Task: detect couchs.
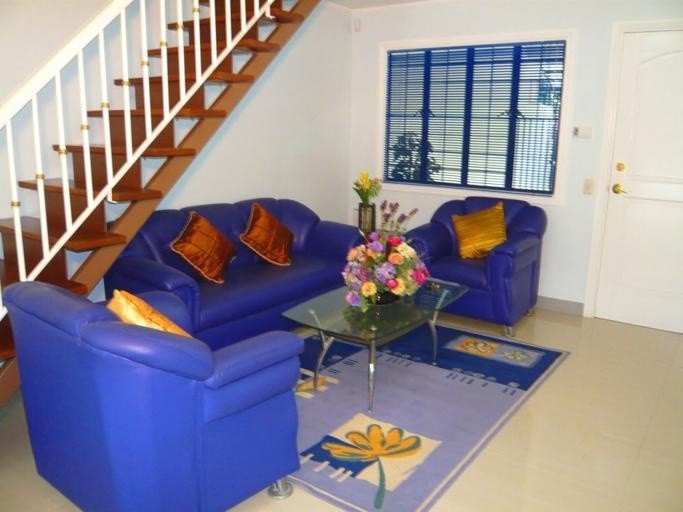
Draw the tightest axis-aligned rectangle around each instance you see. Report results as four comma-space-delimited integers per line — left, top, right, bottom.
102, 198, 364, 351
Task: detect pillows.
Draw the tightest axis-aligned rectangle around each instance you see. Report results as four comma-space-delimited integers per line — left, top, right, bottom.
451, 201, 507, 259
170, 211, 239, 284
238, 202, 295, 266
106, 288, 194, 338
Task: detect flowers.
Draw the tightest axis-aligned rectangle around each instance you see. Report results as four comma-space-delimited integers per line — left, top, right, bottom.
353, 171, 383, 204
340, 200, 430, 312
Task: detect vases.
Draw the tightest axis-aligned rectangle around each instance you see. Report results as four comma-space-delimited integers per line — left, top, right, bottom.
358, 202, 376, 234
366, 291, 400, 304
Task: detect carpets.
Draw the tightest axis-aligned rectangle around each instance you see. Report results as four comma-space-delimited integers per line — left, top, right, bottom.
287, 317, 571, 512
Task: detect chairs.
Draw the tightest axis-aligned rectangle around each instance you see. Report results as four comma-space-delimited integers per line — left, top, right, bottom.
402, 196, 546, 337
2, 281, 305, 512
